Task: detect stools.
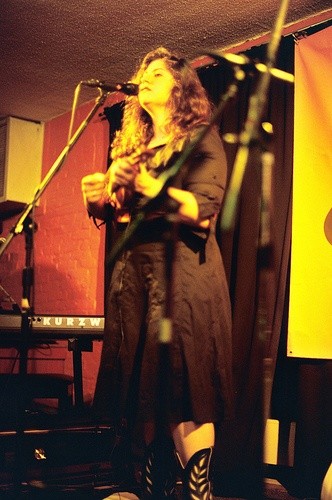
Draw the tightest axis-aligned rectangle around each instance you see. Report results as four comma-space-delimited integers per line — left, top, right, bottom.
5, 376, 73, 499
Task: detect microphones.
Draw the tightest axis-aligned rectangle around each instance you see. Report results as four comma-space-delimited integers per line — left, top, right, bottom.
80, 79, 139, 96
199, 50, 294, 83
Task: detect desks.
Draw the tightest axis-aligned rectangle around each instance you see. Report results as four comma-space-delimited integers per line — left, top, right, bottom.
0, 314, 109, 424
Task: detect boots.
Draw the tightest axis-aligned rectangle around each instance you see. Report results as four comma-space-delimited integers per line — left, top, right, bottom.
173, 448, 212, 500
133, 442, 167, 500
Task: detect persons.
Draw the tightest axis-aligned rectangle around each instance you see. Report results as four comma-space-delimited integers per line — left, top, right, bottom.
82, 48, 228, 500
